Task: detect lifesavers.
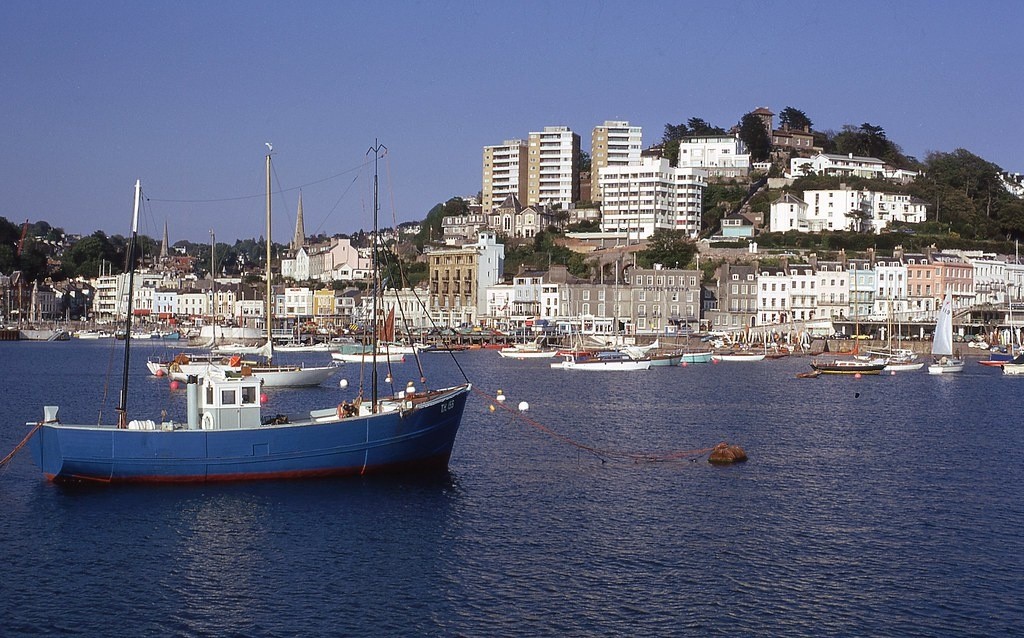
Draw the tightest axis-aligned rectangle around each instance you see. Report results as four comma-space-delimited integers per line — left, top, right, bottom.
201, 412, 213, 430
1001, 365, 1004, 370
170, 362, 179, 372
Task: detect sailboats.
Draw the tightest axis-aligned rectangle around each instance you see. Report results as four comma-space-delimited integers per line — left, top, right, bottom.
53, 143, 471, 389
22, 138, 475, 486
496, 264, 1024, 376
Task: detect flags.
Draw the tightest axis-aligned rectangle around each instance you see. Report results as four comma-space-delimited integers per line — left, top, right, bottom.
379, 305, 395, 343
